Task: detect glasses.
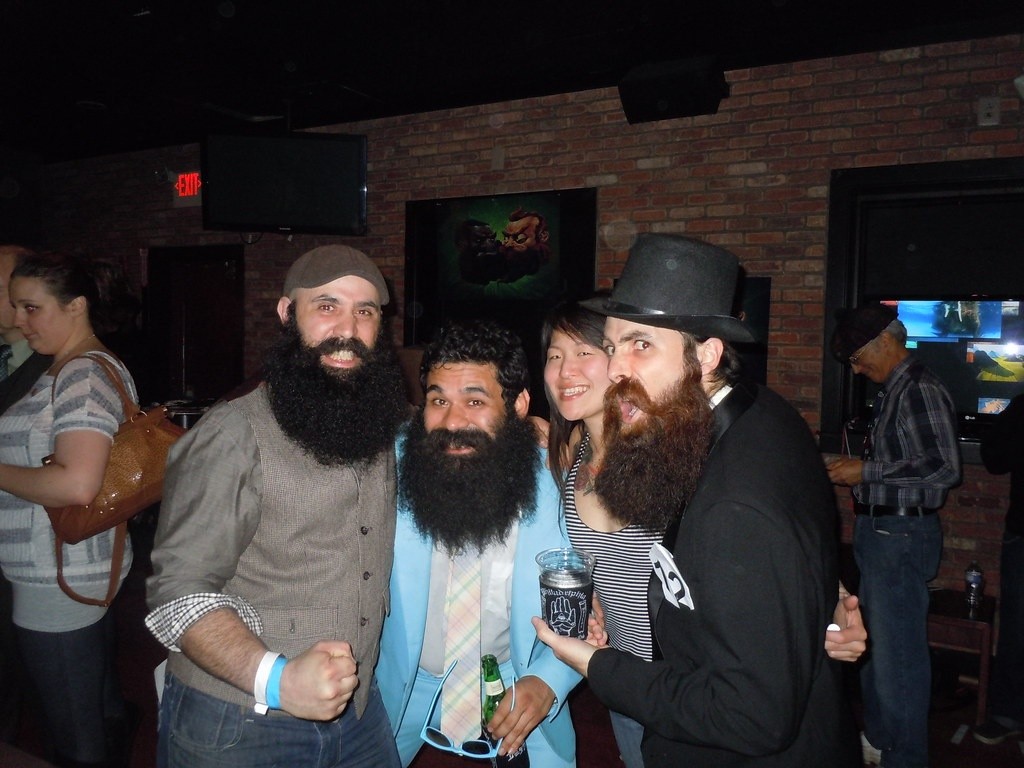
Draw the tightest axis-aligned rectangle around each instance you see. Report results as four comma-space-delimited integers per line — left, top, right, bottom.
419, 660, 516, 760
849, 340, 872, 365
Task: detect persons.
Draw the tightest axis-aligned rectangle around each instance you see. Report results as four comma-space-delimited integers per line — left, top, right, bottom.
0, 242, 132, 768
374, 234, 1024, 768
144, 243, 550, 768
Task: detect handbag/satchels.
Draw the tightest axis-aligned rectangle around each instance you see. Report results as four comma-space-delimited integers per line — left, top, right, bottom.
41, 353, 193, 608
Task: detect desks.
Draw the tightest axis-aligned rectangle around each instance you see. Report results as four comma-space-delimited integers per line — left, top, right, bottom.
928, 589, 997, 727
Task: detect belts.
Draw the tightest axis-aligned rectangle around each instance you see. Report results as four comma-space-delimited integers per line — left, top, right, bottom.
852, 499, 939, 519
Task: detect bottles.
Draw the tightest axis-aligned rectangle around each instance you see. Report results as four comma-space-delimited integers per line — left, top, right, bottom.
963, 559, 985, 606
481, 654, 530, 768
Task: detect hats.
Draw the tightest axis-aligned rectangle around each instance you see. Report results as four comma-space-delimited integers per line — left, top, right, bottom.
576, 231, 757, 345
830, 302, 898, 365
281, 244, 390, 307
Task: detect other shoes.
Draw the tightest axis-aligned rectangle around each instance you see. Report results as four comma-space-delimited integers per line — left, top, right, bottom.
974, 718, 1024, 751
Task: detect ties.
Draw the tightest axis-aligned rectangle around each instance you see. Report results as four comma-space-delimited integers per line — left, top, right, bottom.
0, 345, 13, 384
441, 531, 482, 757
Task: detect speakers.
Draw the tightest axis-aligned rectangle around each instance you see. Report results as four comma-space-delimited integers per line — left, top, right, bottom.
618, 54, 726, 125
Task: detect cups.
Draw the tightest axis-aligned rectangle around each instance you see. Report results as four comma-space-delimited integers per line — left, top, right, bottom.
536, 547, 595, 641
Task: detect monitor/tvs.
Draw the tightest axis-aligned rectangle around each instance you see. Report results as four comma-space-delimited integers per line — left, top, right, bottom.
200, 130, 368, 238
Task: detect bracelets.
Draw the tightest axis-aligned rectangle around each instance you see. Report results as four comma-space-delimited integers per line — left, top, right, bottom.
254, 649, 289, 716
